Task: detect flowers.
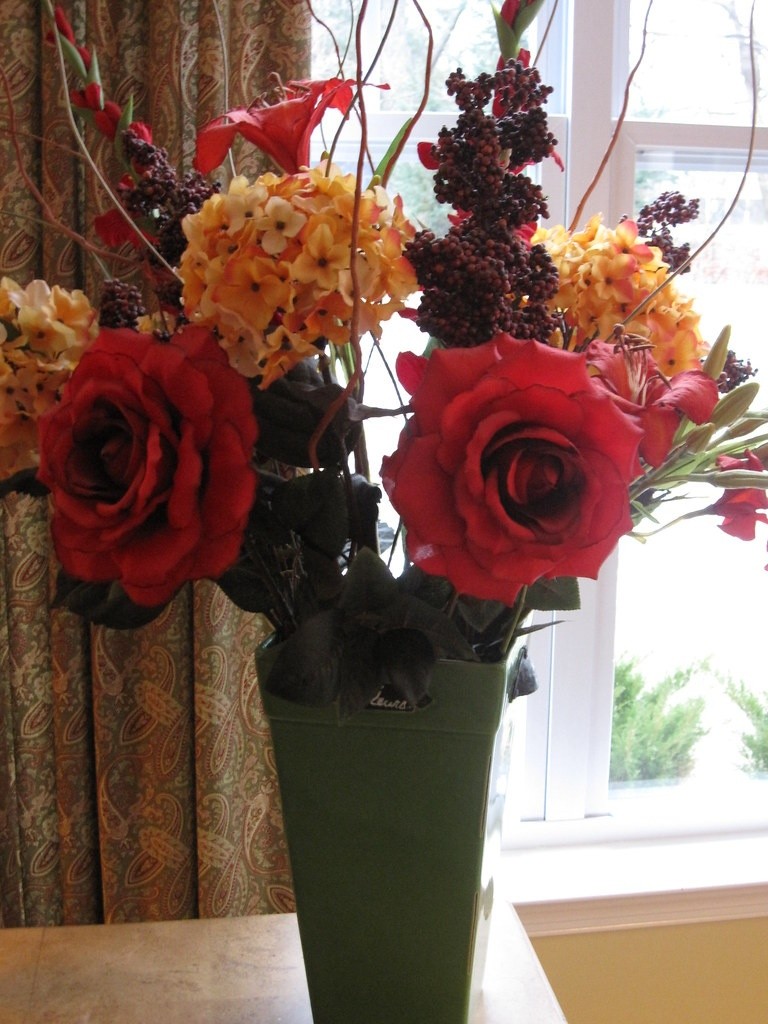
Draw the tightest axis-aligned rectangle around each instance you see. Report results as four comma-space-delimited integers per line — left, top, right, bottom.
0, 0, 768, 711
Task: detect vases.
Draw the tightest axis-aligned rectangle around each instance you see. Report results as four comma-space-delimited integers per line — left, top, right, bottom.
254, 631, 516, 1024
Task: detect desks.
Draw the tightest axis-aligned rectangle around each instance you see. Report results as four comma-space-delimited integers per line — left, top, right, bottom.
0, 899, 572, 1024
